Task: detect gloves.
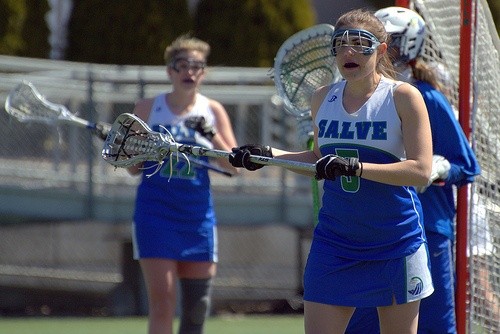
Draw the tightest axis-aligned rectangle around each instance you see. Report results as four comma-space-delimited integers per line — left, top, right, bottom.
184, 115, 217, 138
413, 155, 449, 192
229, 143, 273, 170
314, 153, 359, 180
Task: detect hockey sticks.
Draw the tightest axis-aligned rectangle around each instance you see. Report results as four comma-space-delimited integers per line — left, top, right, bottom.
274, 24, 344, 228
100, 112, 340, 176
4, 81, 233, 176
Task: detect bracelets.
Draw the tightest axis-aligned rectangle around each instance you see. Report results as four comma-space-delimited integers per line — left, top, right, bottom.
358, 163, 363, 177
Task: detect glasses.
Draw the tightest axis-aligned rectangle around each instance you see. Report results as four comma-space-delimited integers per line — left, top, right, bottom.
330, 28, 380, 55
169, 58, 205, 76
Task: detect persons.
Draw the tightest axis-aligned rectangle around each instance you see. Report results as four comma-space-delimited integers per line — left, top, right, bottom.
229, 8, 435, 334
125, 34, 241, 334
343, 7, 481, 334
0, 0, 324, 181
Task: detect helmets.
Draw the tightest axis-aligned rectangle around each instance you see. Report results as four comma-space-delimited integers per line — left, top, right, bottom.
374, 6, 425, 62
